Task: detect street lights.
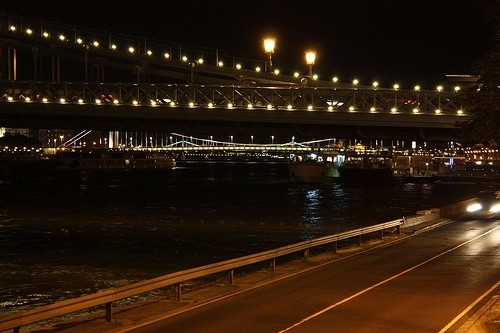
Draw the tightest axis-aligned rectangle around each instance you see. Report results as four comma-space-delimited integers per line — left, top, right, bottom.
306, 51, 315, 80
263, 39, 275, 74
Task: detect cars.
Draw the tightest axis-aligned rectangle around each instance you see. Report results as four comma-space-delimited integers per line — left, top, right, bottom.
468, 194, 500, 219
